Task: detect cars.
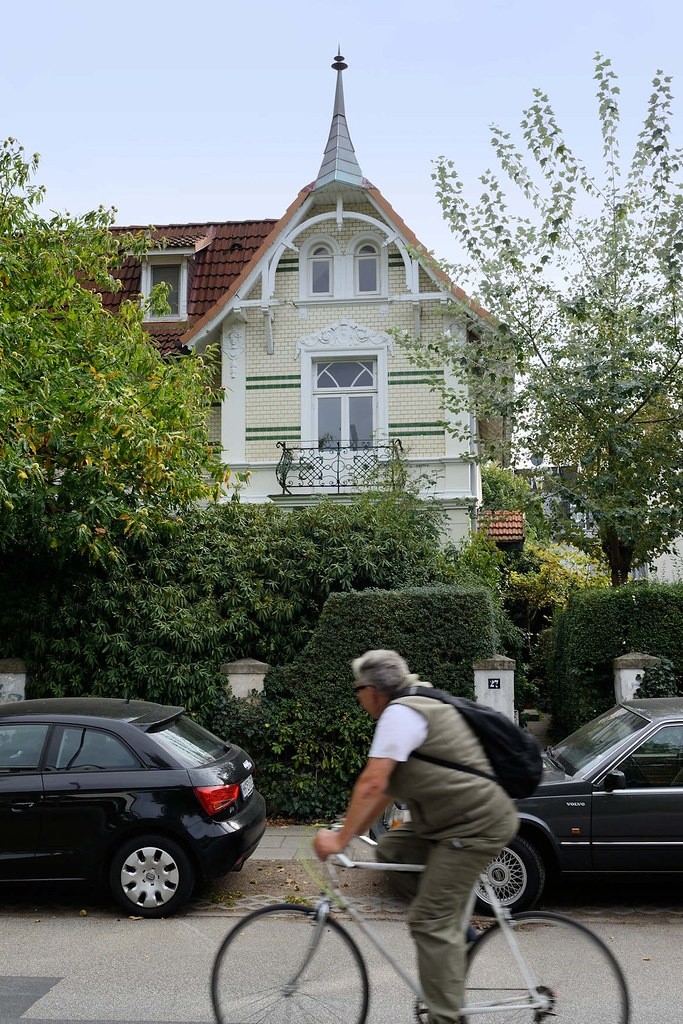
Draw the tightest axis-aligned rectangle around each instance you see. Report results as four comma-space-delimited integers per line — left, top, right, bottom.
369, 694, 683, 918
0, 697, 269, 917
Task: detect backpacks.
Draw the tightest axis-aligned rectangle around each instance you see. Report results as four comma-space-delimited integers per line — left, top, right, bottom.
388, 686, 543, 799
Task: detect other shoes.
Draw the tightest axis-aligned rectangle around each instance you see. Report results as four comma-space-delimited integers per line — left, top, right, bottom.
464, 926, 475, 944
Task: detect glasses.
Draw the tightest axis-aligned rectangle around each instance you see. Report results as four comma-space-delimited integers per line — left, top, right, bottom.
352, 684, 378, 695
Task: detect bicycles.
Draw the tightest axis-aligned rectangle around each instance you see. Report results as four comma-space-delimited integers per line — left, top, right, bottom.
211, 824, 631, 1024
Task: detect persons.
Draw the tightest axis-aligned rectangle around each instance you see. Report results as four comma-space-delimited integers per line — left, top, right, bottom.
312, 651, 517, 1024
